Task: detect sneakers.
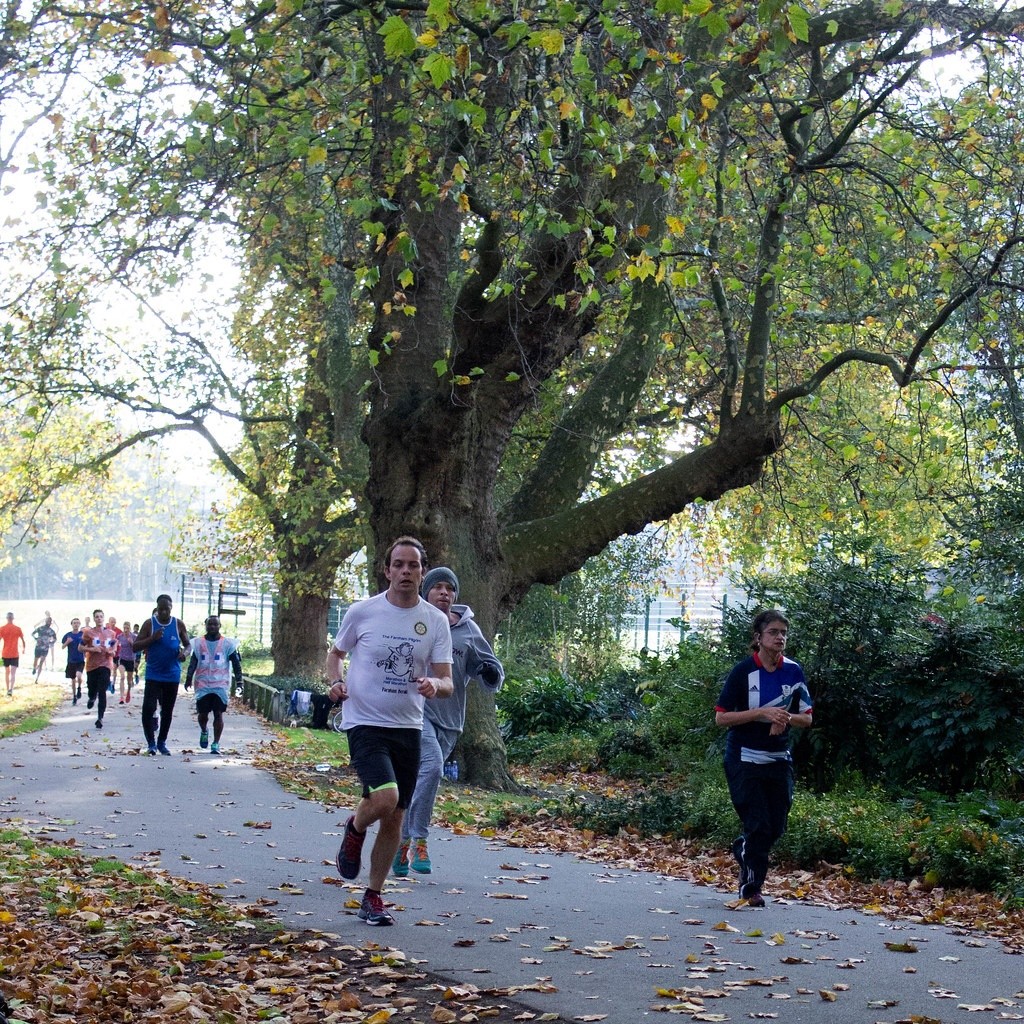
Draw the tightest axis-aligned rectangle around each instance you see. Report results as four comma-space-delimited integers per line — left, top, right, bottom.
748, 892, 765, 906
146, 745, 156, 753
199, 730, 208, 749
357, 892, 397, 926
392, 841, 409, 878
210, 742, 220, 753
409, 838, 431, 874
336, 814, 366, 881
157, 742, 170, 754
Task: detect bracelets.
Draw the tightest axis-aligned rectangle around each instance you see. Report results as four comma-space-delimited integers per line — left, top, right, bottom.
330, 679, 343, 685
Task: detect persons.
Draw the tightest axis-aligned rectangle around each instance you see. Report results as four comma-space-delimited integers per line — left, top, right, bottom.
184, 615, 244, 754
0, 612, 25, 698
32, 607, 160, 729
714, 610, 814, 905
326, 536, 454, 925
132, 595, 191, 755
391, 567, 505, 876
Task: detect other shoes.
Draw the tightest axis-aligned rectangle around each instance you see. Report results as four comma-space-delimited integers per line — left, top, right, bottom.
77, 688, 81, 698
108, 681, 111, 691
135, 675, 139, 684
111, 686, 114, 693
7, 690, 11, 695
72, 696, 77, 705
88, 700, 94, 708
126, 694, 130, 703
95, 720, 103, 728
119, 697, 124, 703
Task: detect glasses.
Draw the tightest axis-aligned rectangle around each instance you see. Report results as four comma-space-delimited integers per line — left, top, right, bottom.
760, 629, 787, 637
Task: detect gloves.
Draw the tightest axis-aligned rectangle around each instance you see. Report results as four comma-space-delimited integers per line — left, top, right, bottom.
479, 663, 502, 686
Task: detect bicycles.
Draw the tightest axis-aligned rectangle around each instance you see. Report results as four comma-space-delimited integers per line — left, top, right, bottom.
332, 710, 347, 734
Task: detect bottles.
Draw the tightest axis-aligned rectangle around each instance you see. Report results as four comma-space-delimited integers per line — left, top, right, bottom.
236, 687, 242, 698
445, 760, 458, 781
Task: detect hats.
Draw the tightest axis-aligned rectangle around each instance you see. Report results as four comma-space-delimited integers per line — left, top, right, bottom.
423, 567, 459, 602
